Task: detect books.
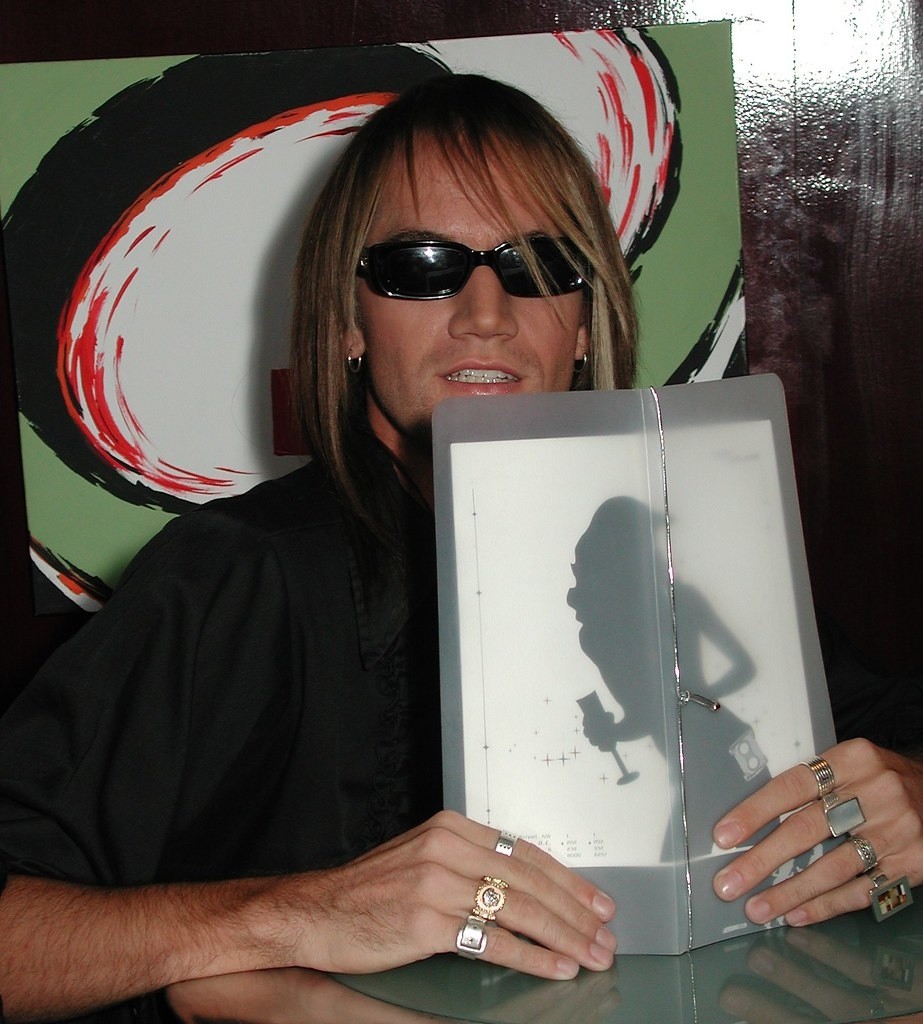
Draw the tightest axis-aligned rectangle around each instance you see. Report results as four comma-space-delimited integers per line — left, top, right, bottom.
433, 374, 849, 956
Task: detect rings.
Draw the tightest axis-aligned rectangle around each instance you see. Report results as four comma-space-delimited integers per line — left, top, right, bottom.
471, 876, 509, 921
872, 945, 914, 991
869, 997, 883, 1020
821, 793, 866, 838
848, 833, 878, 873
798, 756, 834, 797
456, 915, 486, 960
867, 867, 913, 922
495, 831, 519, 856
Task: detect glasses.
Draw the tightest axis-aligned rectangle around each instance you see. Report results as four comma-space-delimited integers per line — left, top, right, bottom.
352, 234, 592, 301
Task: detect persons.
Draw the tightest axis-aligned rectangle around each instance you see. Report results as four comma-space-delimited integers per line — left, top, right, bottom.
1, 76, 923, 1024
